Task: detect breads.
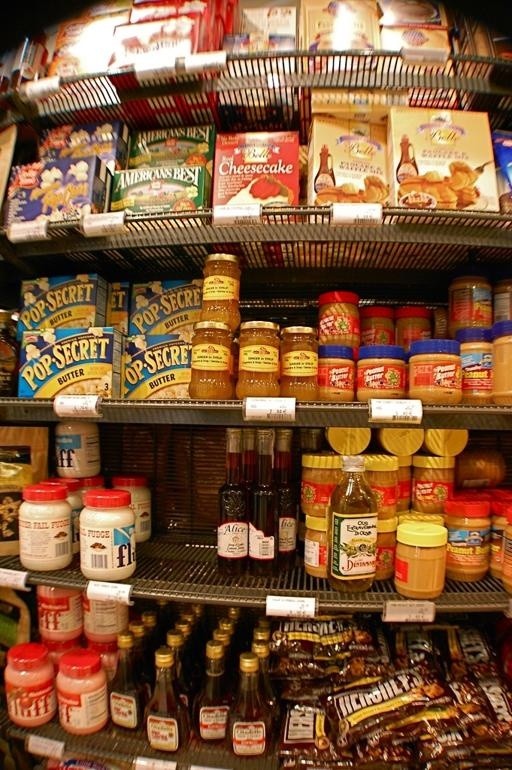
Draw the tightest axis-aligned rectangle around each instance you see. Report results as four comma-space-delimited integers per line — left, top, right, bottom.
398, 162, 477, 208
317, 177, 389, 205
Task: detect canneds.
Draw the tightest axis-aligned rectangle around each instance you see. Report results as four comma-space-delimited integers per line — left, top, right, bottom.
493, 279, 512, 323
201, 254, 242, 331
447, 276, 493, 340
189, 321, 234, 401
236, 320, 280, 401
280, 326, 319, 404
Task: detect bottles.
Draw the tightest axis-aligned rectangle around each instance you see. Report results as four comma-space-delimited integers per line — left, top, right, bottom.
325, 452, 378, 594
216, 424, 315, 578
108, 597, 283, 761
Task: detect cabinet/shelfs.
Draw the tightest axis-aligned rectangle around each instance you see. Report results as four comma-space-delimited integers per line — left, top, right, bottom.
1, 0, 509, 768
